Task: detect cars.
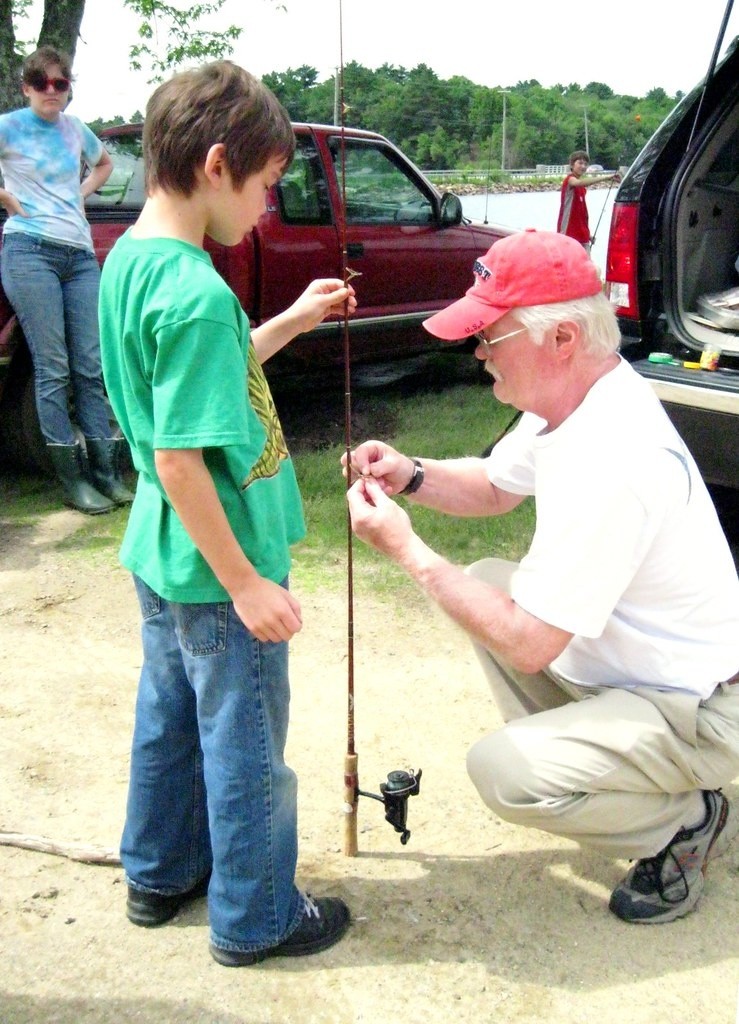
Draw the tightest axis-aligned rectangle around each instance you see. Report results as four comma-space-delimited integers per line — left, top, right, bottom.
601, 0, 739, 493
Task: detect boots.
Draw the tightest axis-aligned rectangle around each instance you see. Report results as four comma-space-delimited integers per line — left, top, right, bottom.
85, 438, 135, 503
46, 440, 118, 514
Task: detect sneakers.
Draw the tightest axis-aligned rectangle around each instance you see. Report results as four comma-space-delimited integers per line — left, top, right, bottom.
210, 892, 350, 968
608, 787, 739, 923
126, 873, 212, 927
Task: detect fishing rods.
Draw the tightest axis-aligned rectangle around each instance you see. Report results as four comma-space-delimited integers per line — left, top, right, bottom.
590, 163, 620, 250
338, 0, 424, 857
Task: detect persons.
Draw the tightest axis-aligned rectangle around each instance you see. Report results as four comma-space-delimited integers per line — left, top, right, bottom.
556, 151, 622, 260
0, 44, 136, 516
340, 228, 739, 925
97, 58, 357, 967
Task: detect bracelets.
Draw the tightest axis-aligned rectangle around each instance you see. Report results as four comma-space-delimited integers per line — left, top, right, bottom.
398, 457, 425, 496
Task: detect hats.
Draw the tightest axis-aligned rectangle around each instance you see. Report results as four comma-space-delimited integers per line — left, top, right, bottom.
421, 229, 602, 341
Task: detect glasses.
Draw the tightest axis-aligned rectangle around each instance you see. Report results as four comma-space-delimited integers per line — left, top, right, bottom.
26, 78, 70, 92
475, 327, 527, 359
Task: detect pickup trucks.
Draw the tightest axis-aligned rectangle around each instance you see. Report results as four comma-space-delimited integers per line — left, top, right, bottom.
0, 119, 517, 477
